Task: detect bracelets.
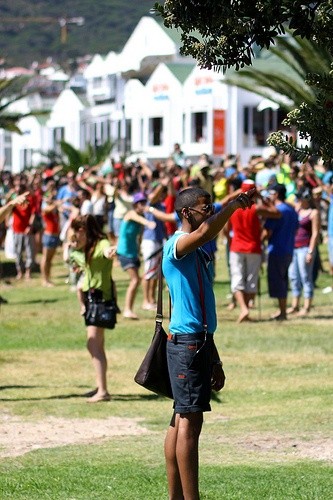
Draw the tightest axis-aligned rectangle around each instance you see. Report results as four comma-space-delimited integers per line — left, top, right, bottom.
307, 250, 312, 254
230, 199, 240, 210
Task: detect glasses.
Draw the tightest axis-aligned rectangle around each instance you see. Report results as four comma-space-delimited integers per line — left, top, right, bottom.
203, 204, 216, 214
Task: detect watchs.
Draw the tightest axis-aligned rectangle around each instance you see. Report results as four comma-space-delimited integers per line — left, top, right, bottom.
148, 206, 153, 213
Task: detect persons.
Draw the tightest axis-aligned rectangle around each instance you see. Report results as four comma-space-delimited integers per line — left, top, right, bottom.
68, 214, 117, 403
117, 193, 156, 319
286, 187, 321, 317
161, 187, 257, 500
142, 182, 167, 310
0, 143, 333, 291
259, 184, 300, 319
222, 180, 282, 324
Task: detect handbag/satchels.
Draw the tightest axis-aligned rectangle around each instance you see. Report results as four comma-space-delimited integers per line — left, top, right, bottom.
85, 301, 117, 329
136, 321, 172, 399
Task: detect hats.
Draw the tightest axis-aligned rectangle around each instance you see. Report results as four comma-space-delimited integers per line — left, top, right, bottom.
132, 193, 147, 205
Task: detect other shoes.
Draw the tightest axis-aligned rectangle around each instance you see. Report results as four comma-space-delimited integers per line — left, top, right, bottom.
123, 310, 138, 319
86, 390, 96, 397
237, 310, 248, 323
274, 314, 287, 321
142, 300, 157, 310
88, 394, 110, 402
